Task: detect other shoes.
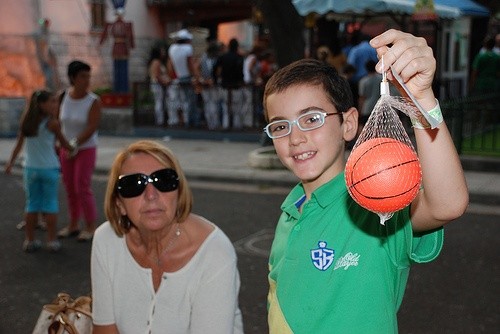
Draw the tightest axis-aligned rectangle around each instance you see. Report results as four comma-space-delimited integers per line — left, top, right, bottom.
58, 228, 78, 237
78, 232, 92, 240
48, 241, 60, 252
24, 240, 41, 252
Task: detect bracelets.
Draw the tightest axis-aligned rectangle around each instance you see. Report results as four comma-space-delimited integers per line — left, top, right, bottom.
410, 98, 443, 129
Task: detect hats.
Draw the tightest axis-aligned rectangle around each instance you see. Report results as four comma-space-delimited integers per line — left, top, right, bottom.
169, 29, 194, 39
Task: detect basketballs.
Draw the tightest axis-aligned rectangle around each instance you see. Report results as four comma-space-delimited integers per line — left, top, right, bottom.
344, 138, 421, 212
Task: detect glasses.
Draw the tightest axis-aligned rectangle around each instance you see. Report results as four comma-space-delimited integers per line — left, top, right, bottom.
116, 168, 179, 198
264, 112, 343, 139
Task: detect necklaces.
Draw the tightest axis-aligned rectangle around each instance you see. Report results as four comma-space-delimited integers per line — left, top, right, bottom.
140, 223, 180, 260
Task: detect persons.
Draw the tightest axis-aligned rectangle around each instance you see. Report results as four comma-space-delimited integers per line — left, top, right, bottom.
49, 60, 102, 241
99, 0, 135, 93
4, 90, 74, 252
91, 141, 244, 334
304, 30, 463, 145
264, 30, 469, 334
145, 28, 281, 130
37, 18, 64, 94
468, 32, 500, 128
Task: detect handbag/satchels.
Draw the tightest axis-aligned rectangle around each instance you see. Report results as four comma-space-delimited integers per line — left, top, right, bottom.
33, 294, 93, 334
